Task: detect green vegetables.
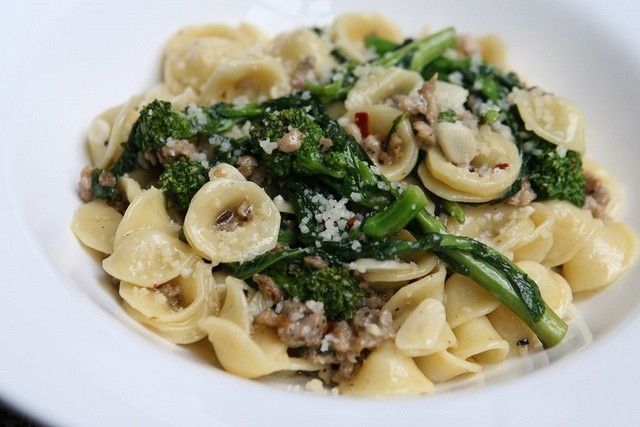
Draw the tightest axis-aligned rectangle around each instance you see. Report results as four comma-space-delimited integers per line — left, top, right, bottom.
90, 25, 585, 350
